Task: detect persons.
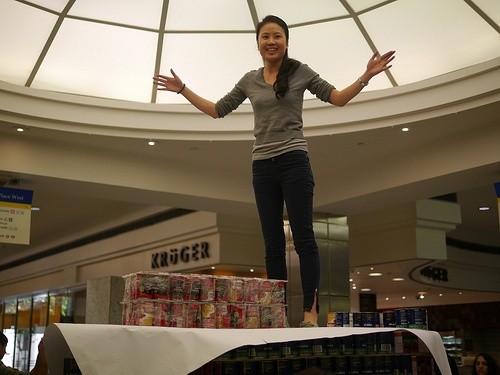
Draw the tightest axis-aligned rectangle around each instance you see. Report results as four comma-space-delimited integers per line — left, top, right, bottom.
446, 350, 459, 375
0, 332, 24, 375
153, 15, 395, 328
472, 353, 500, 375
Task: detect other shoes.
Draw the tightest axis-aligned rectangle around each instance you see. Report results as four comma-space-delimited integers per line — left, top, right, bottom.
299, 321, 320, 327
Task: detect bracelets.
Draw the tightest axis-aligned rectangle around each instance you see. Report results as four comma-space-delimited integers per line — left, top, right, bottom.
358, 78, 368, 87
177, 84, 185, 94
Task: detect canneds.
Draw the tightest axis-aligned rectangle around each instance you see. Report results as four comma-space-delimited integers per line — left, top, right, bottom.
121, 271, 286, 328
187, 329, 432, 375
334, 309, 428, 330
